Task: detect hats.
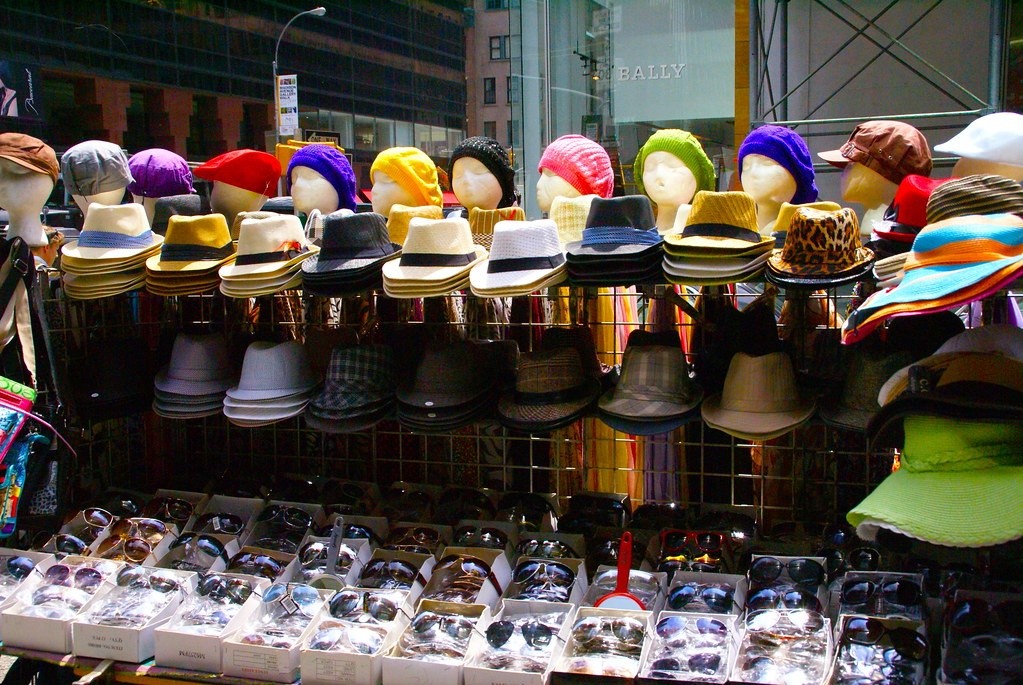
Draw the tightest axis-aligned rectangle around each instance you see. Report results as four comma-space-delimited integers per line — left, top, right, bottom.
60, 191, 1023, 548
127, 148, 196, 198
193, 149, 282, 198
539, 134, 614, 198
447, 137, 516, 210
634, 127, 716, 206
0, 131, 59, 184
816, 119, 933, 186
739, 126, 819, 204
285, 144, 356, 212
936, 112, 1022, 168
60, 141, 137, 196
370, 147, 444, 209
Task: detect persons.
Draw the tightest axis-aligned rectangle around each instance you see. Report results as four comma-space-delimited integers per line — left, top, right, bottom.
818, 119, 935, 211
448, 136, 517, 215
192, 149, 282, 234
127, 148, 194, 228
536, 134, 615, 212
934, 111, 1023, 183
60, 139, 135, 222
738, 123, 819, 235
287, 144, 357, 220
0, 132, 61, 247
633, 127, 718, 232
370, 146, 444, 220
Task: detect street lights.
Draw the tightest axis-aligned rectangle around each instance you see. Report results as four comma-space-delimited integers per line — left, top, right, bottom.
271, 7, 328, 147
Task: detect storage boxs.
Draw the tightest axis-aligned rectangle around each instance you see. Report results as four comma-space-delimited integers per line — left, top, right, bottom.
1, 487, 1023, 685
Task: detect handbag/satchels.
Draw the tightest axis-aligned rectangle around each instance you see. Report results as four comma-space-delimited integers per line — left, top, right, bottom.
0, 236, 60, 542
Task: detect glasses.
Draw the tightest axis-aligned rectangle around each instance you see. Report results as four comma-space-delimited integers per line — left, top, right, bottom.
0, 487, 1023, 685
